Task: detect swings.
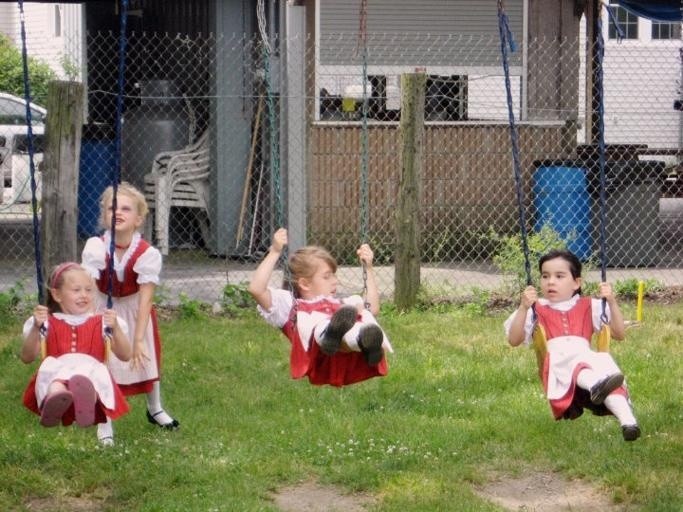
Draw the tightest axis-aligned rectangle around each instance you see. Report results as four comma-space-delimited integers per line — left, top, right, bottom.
18, 1, 113, 366
258, 0, 393, 353
498, 2, 614, 367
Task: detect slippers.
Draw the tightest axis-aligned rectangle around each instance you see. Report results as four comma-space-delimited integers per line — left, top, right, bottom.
40, 376, 98, 427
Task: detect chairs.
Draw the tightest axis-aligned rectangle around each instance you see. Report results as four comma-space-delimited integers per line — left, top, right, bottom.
142, 126, 210, 256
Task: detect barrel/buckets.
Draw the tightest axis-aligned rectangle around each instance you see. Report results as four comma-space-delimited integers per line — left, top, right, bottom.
76, 77, 192, 251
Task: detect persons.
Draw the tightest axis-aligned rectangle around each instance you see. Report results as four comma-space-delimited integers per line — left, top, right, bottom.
19, 263, 133, 430
503, 252, 641, 442
80, 182, 180, 446
247, 228, 393, 388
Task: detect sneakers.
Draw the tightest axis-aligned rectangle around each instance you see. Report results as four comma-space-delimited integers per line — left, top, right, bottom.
590, 374, 624, 405
322, 307, 355, 355
622, 424, 640, 441
360, 326, 383, 363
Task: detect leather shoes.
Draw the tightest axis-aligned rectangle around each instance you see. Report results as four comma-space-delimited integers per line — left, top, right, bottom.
146, 407, 180, 429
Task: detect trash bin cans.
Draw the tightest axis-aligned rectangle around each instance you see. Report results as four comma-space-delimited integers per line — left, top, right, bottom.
11, 134, 45, 203
78, 123, 120, 241
589, 161, 666, 267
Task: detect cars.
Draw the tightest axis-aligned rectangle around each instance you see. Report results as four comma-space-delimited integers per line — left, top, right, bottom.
0, 93, 47, 185
